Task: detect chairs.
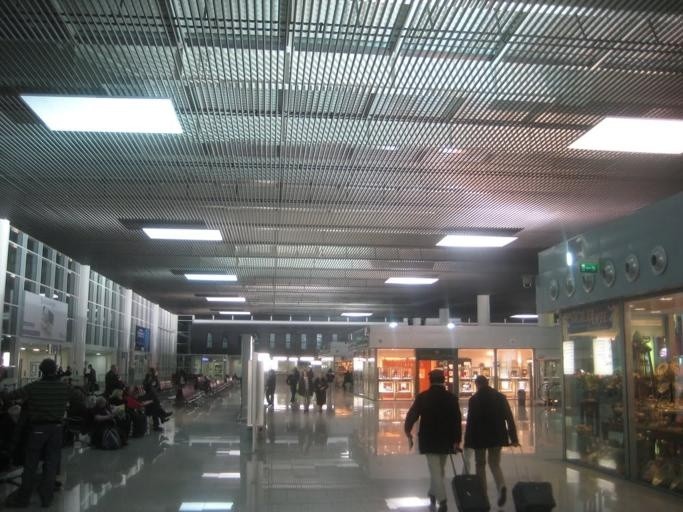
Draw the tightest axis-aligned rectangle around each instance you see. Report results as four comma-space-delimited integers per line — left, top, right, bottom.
133, 377, 229, 410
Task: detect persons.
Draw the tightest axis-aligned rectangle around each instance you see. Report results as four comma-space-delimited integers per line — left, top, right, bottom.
0, 356, 187, 511
286, 366, 355, 413
404, 369, 463, 512
264, 369, 275, 409
463, 375, 520, 507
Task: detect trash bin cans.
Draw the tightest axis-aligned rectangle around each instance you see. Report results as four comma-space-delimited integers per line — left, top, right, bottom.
518, 390, 525, 407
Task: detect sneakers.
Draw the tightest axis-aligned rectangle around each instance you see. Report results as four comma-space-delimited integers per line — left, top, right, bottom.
4, 498, 30, 507
497, 484, 508, 507
153, 411, 172, 432
427, 493, 448, 510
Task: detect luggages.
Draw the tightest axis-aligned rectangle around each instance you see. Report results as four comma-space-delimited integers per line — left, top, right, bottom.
511, 481, 555, 511
132, 414, 148, 439
452, 474, 491, 511
